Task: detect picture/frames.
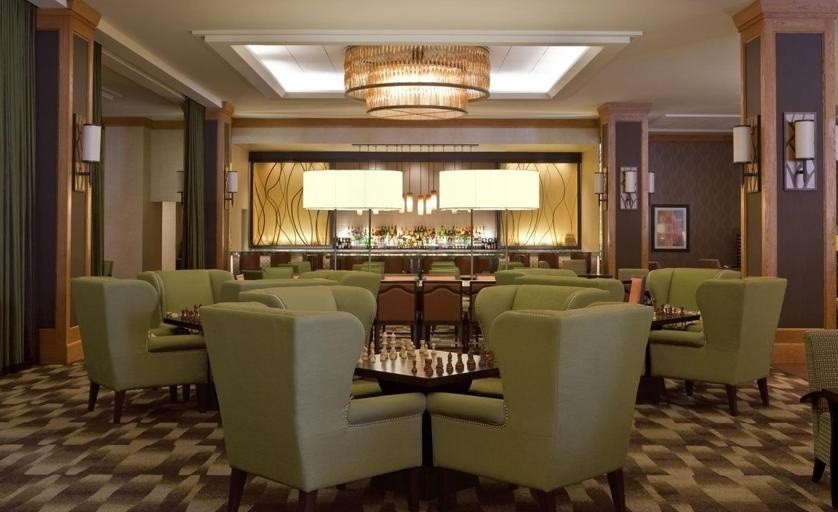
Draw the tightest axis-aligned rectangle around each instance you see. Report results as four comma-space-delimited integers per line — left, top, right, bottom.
650, 201, 691, 252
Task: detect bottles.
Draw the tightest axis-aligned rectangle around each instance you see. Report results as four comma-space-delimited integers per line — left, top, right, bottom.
333, 224, 499, 250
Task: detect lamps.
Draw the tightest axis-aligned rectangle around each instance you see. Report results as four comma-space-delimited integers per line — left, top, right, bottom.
731, 118, 818, 185
222, 167, 240, 208
591, 168, 658, 209
339, 45, 494, 123
172, 167, 188, 204
73, 118, 102, 188
301, 145, 542, 280
